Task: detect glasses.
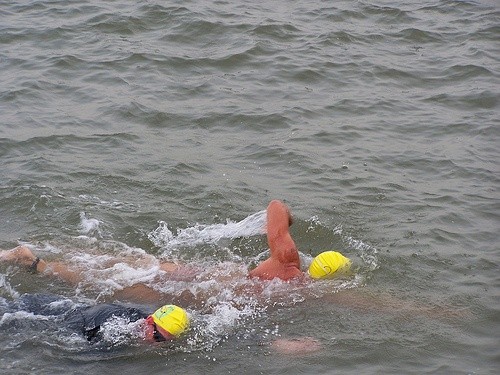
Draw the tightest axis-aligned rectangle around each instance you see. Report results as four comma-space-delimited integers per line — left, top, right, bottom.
153, 322, 166, 342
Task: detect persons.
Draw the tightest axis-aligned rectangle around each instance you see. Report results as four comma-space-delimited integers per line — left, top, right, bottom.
0, 200, 350, 302
0, 295, 321, 360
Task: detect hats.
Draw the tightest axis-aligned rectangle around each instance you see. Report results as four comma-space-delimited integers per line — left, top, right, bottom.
152, 304, 190, 338
308, 250, 352, 280
